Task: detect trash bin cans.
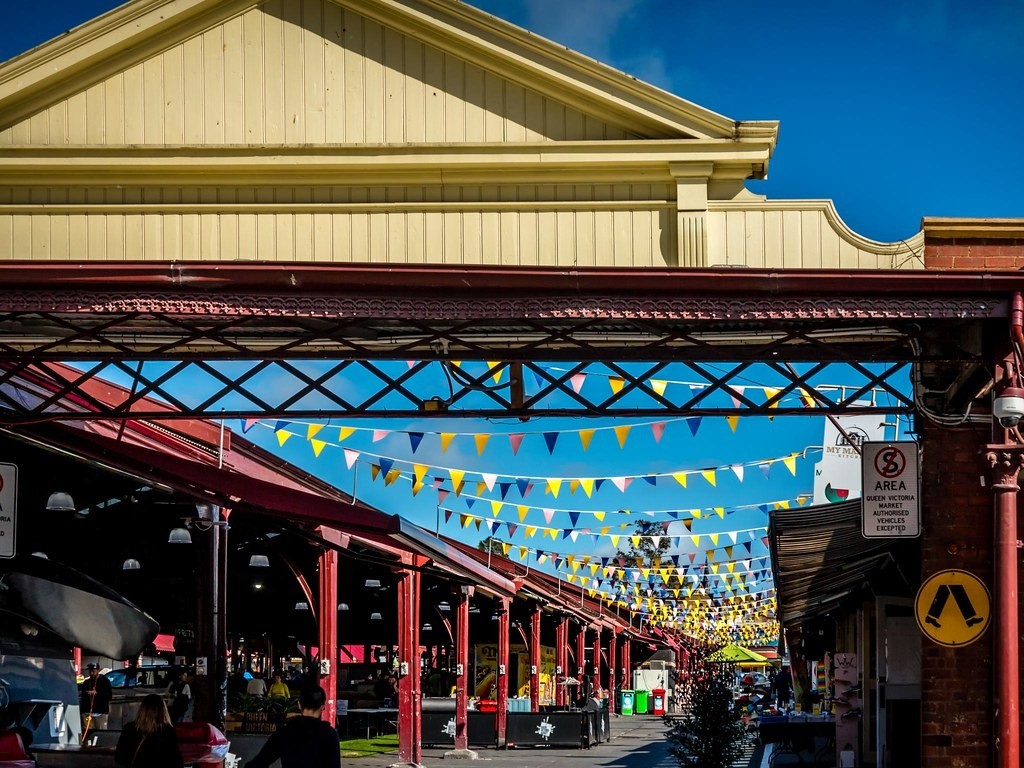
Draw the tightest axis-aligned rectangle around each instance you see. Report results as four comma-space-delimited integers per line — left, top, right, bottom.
652, 689, 666, 715
621, 690, 635, 716
635, 689, 650, 715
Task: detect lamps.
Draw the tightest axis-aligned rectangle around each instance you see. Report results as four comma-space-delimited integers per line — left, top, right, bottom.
820, 588, 852, 604
25, 490, 524, 631
423, 400, 442, 412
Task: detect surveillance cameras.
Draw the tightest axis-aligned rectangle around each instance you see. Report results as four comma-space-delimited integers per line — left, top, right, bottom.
993, 397, 1024, 427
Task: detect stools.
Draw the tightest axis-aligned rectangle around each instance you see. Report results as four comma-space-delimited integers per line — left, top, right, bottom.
768, 731, 835, 768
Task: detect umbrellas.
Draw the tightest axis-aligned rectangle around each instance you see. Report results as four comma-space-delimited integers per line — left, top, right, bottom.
701, 645, 768, 684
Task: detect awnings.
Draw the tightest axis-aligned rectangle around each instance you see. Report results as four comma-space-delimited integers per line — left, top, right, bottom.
769, 495, 918, 658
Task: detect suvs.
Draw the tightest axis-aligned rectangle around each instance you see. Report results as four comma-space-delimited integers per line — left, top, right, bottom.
78, 665, 199, 698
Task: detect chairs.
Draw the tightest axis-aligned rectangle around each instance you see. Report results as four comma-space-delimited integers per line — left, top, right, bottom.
353, 696, 373, 708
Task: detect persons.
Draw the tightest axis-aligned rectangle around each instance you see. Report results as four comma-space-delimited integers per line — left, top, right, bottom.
266, 675, 291, 700
579, 673, 594, 704
247, 673, 267, 699
768, 671, 775, 681
773, 666, 793, 709
243, 683, 341, 768
81, 662, 112, 731
115, 694, 185, 768
379, 675, 399, 707
227, 668, 249, 713
167, 671, 192, 722
738, 685, 769, 714
246, 666, 305, 681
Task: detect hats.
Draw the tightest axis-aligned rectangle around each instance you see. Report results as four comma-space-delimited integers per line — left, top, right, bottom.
86, 663, 100, 670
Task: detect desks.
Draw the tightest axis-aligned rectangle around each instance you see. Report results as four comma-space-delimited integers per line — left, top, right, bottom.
760, 716, 837, 755
337, 690, 361, 702
356, 699, 388, 742
347, 708, 401, 741
